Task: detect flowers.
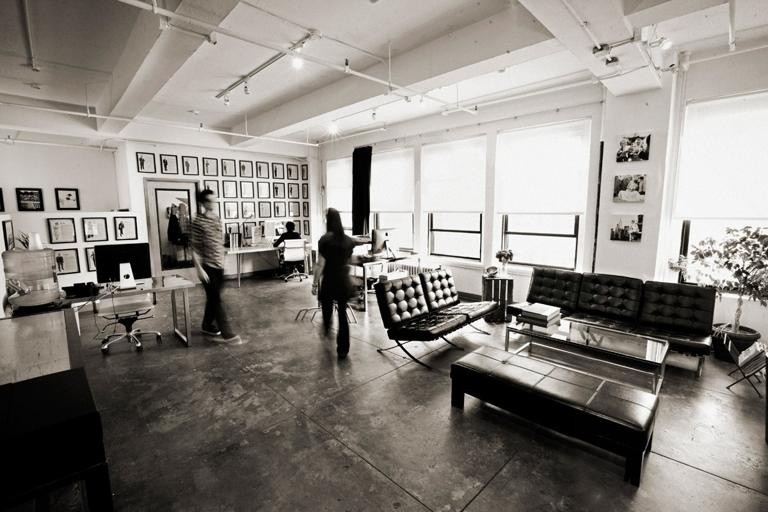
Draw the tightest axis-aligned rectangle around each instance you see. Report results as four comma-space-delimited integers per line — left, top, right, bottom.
494, 249, 512, 263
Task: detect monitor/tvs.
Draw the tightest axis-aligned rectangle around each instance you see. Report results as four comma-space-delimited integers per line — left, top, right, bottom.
94, 242, 152, 289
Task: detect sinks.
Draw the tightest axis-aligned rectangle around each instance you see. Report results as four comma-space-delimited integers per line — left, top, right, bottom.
8, 283, 61, 306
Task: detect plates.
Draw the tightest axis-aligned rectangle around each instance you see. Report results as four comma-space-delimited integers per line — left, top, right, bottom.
486, 266, 497, 275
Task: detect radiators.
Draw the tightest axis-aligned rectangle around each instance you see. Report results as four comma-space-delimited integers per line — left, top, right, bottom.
380, 262, 438, 276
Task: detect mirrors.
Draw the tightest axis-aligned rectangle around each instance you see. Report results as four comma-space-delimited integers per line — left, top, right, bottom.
155, 187, 194, 270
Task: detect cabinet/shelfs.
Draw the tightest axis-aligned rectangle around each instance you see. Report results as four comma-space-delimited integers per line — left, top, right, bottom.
0, 366, 115, 512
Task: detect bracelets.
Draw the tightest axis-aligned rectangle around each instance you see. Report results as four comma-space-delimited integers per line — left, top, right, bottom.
312, 284, 317, 288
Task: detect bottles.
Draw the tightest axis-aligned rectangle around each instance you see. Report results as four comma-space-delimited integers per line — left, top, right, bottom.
27, 231, 42, 251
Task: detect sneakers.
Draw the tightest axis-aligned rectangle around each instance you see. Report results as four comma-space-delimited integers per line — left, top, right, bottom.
223, 333, 238, 341
200, 327, 221, 336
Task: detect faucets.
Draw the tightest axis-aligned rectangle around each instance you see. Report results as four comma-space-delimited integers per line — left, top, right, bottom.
6, 279, 26, 295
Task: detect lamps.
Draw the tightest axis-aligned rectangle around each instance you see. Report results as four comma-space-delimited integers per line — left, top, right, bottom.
221, 75, 251, 108
648, 35, 672, 49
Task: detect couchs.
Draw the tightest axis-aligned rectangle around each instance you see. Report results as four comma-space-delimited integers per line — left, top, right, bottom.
508, 267, 715, 377
374, 268, 498, 373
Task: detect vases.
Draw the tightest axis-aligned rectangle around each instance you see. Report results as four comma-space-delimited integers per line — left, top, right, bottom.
500, 263, 506, 276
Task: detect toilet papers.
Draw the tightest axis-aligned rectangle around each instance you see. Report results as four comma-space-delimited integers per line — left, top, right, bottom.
28, 232, 44, 251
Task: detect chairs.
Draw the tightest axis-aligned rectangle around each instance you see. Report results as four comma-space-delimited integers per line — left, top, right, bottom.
276, 238, 309, 282
92, 241, 166, 352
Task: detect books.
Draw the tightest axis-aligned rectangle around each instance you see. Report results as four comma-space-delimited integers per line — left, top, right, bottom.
517, 302, 563, 328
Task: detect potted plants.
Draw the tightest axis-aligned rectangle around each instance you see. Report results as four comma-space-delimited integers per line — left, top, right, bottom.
667, 222, 767, 363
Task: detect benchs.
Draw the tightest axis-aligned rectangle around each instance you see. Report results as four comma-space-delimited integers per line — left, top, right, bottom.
448, 342, 659, 486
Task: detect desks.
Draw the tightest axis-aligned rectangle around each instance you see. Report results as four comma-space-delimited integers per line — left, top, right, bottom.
346, 248, 421, 311
224, 241, 312, 288
0, 275, 194, 349
503, 317, 667, 400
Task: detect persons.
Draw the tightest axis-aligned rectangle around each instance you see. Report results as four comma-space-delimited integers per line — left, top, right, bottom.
311, 207, 355, 359
627, 220, 639, 241
136, 151, 310, 238
274, 221, 302, 279
90, 251, 96, 267
119, 221, 125, 236
618, 176, 645, 202
53, 221, 61, 241
56, 253, 64, 271
617, 134, 649, 161
188, 189, 239, 342
614, 224, 623, 239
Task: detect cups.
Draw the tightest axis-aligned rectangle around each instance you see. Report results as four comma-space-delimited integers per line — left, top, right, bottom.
230, 233, 239, 249
251, 226, 262, 242
264, 219, 276, 237
144, 279, 152, 289
153, 276, 164, 287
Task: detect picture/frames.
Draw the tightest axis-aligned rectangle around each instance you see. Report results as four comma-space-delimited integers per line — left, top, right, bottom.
83, 217, 109, 242
15, 187, 45, 212
84, 247, 96, 272
54, 248, 80, 274
135, 151, 309, 239
113, 216, 138, 241
47, 217, 77, 244
54, 187, 81, 211
2, 220, 16, 251
